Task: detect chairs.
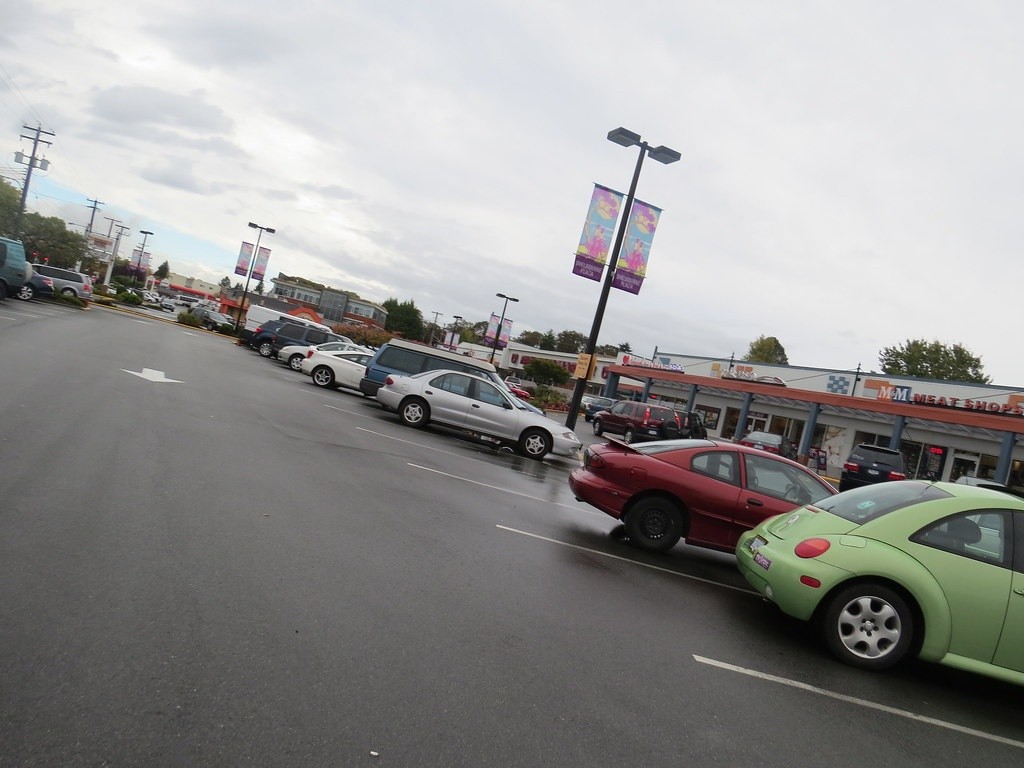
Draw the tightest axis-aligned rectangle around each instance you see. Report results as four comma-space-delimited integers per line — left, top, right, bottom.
728, 460, 758, 490
926, 518, 982, 554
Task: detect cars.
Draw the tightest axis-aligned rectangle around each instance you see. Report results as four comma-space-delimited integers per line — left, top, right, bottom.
740, 430, 799, 466
16, 270, 56, 302
503, 376, 530, 399
673, 410, 708, 440
735, 479, 1024, 686
249, 320, 375, 373
300, 350, 373, 392
377, 369, 583, 460
136, 287, 236, 333
933, 475, 1004, 560
568, 428, 843, 556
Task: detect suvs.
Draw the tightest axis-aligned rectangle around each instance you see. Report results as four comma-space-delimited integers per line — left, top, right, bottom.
591, 401, 683, 444
838, 441, 907, 490
0, 236, 33, 302
571, 393, 620, 421
357, 336, 545, 417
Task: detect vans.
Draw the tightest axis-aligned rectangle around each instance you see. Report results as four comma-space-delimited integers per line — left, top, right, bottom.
242, 305, 332, 350
32, 264, 94, 303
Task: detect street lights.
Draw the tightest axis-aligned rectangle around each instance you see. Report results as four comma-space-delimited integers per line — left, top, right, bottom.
489, 293, 519, 361
565, 125, 679, 426
233, 222, 276, 330
450, 316, 461, 351
131, 230, 154, 289
